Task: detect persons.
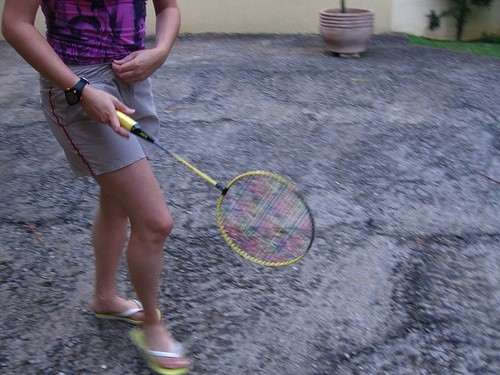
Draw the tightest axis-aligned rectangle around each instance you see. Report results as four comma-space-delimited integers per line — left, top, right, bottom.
1, 0, 192, 375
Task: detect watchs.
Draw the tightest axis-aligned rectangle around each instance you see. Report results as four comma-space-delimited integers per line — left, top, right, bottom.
63, 76, 90, 106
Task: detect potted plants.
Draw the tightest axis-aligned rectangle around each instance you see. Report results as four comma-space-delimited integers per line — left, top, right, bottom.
320, 0, 374, 57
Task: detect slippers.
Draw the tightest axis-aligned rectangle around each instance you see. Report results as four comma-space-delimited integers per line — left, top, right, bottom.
95, 299, 160, 324
129, 326, 190, 375
